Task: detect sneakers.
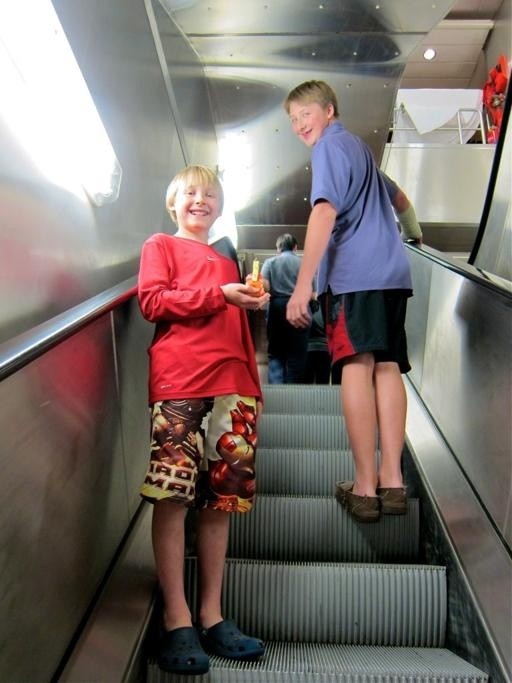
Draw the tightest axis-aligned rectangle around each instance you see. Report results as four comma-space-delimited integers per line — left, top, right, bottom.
334, 480, 408, 524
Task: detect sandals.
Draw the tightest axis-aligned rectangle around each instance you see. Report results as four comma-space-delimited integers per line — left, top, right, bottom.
197, 619, 264, 661
157, 626, 209, 676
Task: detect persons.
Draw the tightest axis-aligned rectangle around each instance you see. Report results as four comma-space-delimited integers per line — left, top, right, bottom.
137, 161, 274, 674
245, 231, 316, 384
284, 79, 423, 522
267, 302, 358, 385
